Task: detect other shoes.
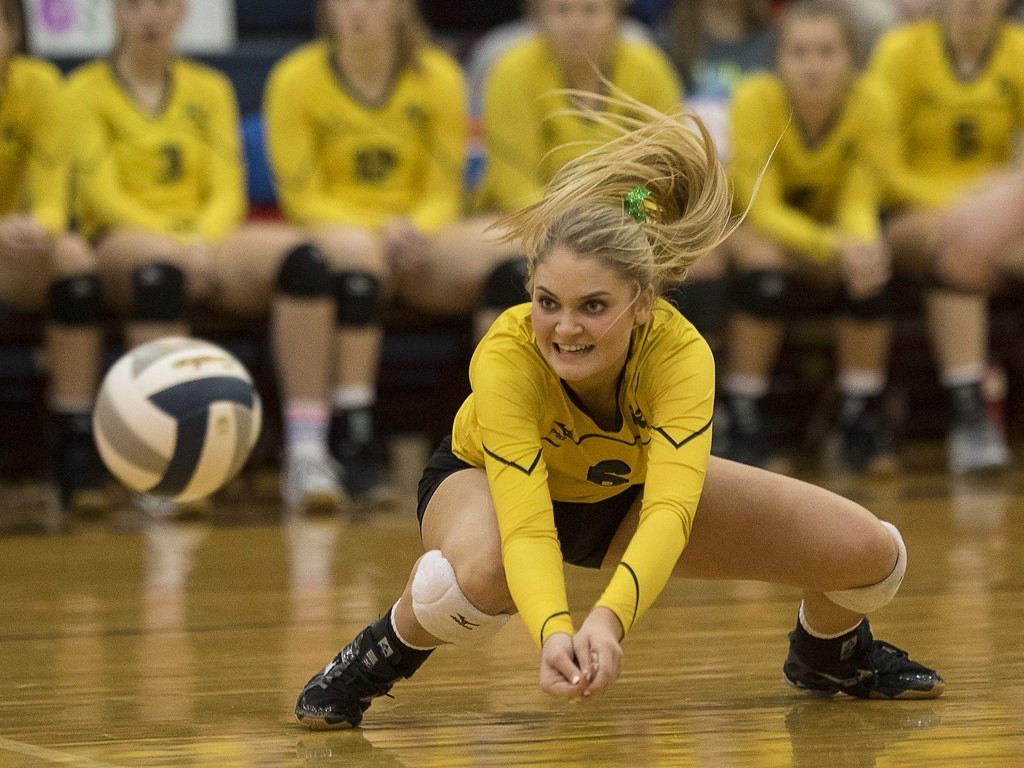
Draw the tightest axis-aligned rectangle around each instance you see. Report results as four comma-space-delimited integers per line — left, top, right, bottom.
277, 449, 348, 516
0, 428, 60, 510
331, 401, 396, 513
723, 390, 793, 476
50, 433, 124, 510
836, 383, 904, 476
947, 417, 1012, 477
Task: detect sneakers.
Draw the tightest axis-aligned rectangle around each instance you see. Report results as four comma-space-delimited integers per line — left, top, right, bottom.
784, 694, 942, 768
781, 618, 945, 699
294, 617, 435, 729
298, 729, 404, 768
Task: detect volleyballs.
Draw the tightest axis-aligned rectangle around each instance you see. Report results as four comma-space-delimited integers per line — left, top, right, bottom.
92, 336, 262, 504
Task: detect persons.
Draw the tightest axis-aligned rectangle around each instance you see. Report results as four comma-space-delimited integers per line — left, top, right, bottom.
293, 64, 945, 731
0, 0, 1023, 542
